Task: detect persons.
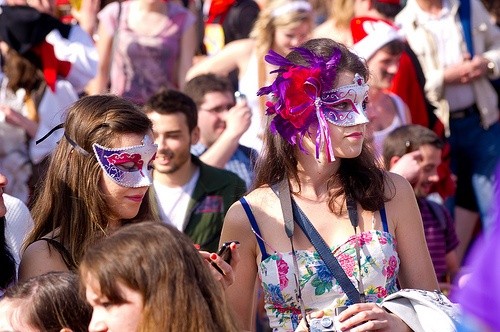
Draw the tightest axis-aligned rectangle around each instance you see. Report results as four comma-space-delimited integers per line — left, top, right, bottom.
77, 221, 248, 332
17, 94, 241, 290
0, 0, 500, 286
217, 39, 442, 332
0, 270, 93, 332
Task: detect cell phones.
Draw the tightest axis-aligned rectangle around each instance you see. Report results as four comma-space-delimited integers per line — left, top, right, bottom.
406, 141, 411, 154
211, 240, 240, 272
235, 92, 246, 104
